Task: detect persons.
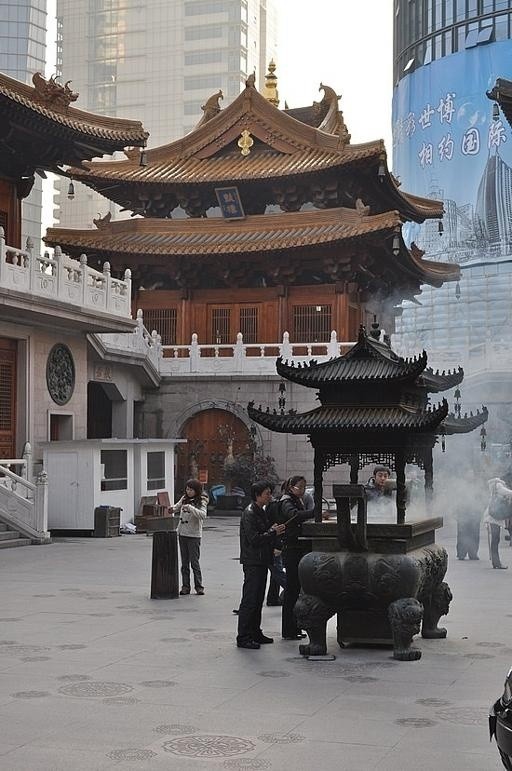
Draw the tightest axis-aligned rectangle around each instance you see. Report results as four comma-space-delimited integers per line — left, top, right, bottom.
166, 479, 211, 596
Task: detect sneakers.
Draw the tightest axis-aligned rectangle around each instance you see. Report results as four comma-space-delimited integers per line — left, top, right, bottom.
236, 630, 274, 649
179, 586, 205, 596
267, 599, 284, 606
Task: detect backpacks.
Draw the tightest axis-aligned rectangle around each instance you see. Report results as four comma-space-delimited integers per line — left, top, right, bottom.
265, 497, 299, 550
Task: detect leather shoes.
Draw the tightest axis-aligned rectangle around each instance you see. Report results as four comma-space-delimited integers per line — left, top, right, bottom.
283, 632, 306, 641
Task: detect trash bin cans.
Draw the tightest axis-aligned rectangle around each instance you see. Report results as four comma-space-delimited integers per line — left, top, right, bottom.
95, 505, 123, 537
